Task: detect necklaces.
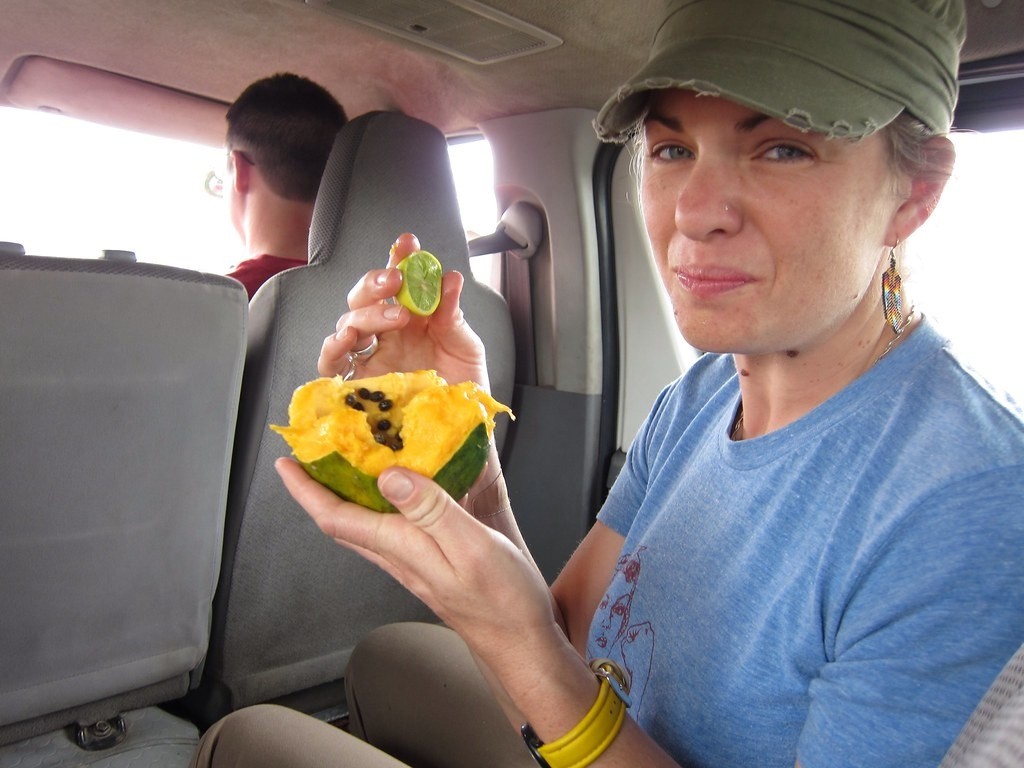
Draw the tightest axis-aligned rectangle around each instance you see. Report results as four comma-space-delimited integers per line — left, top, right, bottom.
732, 305, 916, 445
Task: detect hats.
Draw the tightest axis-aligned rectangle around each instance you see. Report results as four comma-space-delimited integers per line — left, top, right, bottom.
594, 0, 964, 146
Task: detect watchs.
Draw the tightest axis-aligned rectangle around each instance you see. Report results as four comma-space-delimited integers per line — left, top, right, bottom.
520, 658, 632, 768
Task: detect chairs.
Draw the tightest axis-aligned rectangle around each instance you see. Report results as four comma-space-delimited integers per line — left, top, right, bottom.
0, 241, 251, 752
173, 109, 516, 742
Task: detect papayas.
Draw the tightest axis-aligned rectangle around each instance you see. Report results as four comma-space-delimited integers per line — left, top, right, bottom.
269, 369, 516, 514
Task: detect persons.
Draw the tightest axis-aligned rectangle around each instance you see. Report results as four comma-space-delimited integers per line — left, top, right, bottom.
223, 73, 348, 304
192, 1, 1024, 768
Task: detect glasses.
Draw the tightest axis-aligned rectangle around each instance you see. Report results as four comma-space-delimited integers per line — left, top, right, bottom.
206, 150, 255, 197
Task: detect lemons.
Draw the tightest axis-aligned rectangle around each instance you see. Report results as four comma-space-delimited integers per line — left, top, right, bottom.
394, 250, 442, 317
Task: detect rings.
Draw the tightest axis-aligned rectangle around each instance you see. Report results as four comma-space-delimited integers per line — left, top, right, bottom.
343, 362, 356, 381
349, 335, 379, 361
